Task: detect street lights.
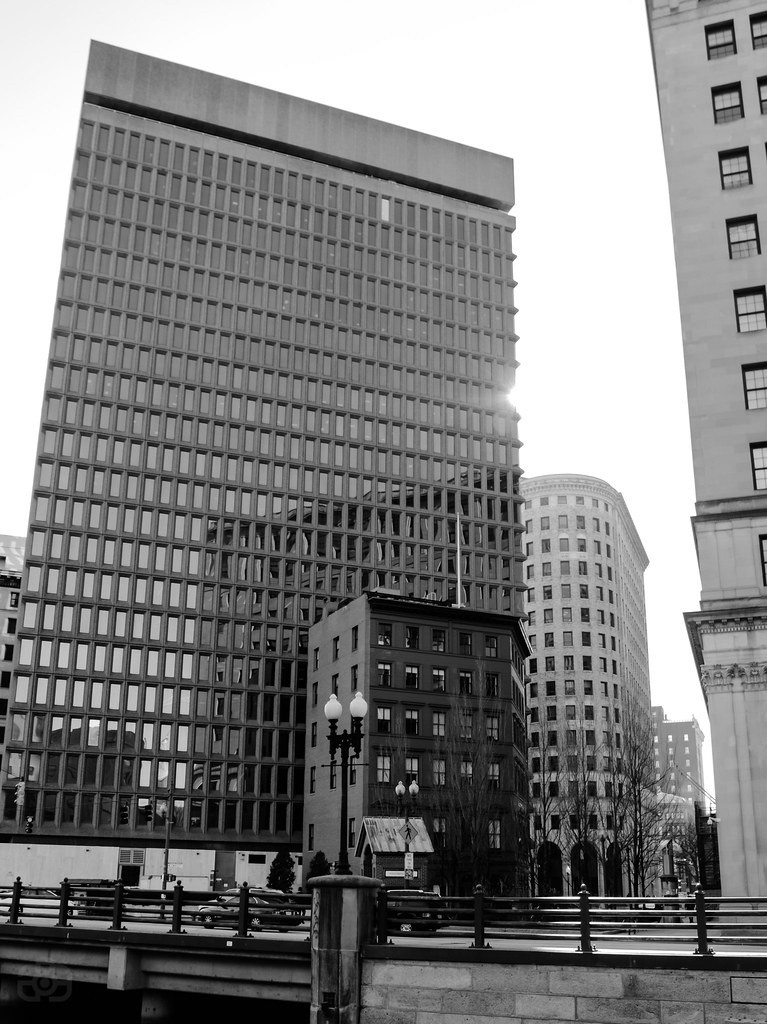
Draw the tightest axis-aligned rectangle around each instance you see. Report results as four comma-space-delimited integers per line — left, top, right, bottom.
396, 779, 420, 890
325, 691, 368, 875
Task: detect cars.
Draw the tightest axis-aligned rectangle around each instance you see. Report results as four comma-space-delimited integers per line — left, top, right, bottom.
199, 888, 302, 933
386, 889, 451, 932
